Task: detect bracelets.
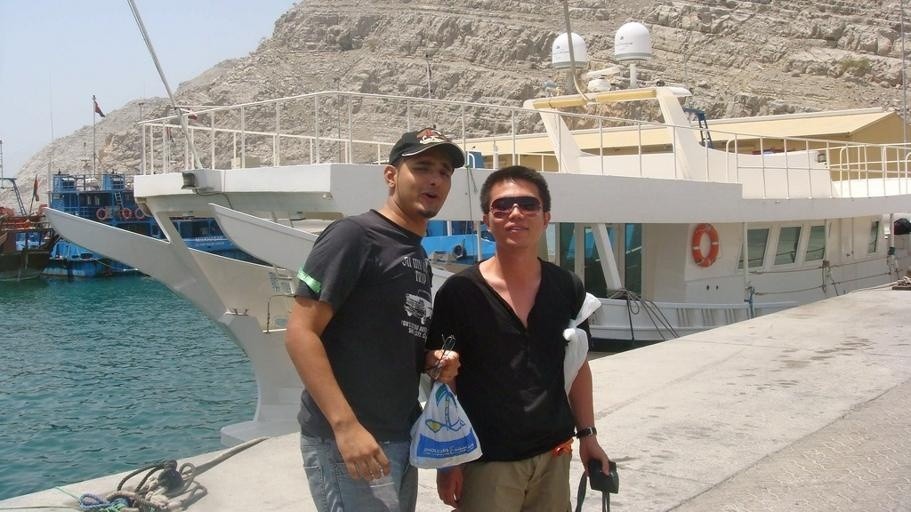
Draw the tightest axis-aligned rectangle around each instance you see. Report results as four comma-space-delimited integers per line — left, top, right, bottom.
576, 425, 597, 439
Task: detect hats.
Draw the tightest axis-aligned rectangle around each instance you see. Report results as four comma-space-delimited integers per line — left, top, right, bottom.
389, 128, 465, 168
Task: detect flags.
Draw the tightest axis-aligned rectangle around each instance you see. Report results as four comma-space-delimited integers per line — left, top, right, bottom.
35, 179, 39, 202
187, 107, 198, 121
94, 99, 104, 118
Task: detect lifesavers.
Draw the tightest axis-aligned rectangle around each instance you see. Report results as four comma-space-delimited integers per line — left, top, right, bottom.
135, 208, 145, 220
122, 207, 132, 219
96, 208, 107, 221
692, 223, 719, 267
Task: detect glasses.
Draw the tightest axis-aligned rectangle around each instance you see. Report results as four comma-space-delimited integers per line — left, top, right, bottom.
488, 196, 540, 217
422, 334, 457, 380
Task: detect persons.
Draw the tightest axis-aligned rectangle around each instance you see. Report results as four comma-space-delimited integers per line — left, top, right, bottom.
427, 166, 613, 512
283, 130, 459, 511
